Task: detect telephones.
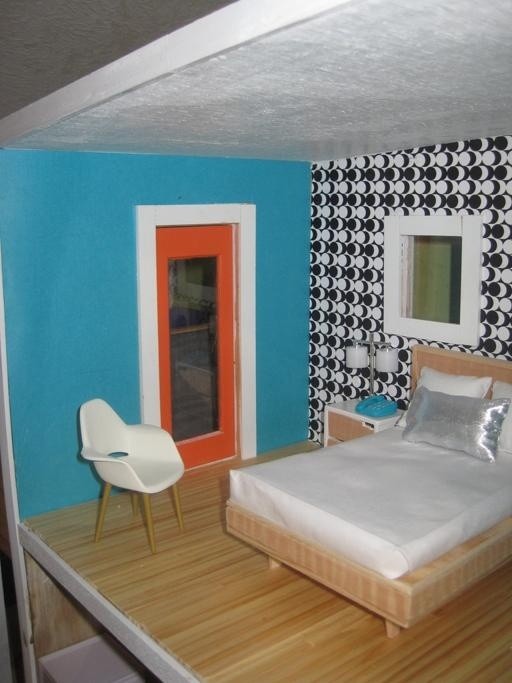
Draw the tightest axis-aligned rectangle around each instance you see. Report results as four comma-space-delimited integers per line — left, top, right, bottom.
355, 396, 398, 417
